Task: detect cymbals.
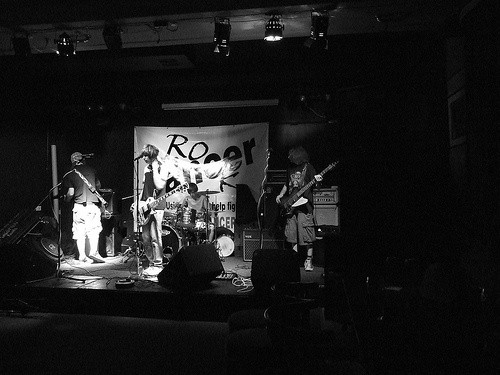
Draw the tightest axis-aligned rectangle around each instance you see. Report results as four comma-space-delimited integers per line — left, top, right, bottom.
195, 191, 220, 194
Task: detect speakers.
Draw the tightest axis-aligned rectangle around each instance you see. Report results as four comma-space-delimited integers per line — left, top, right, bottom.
242, 228, 301, 294
157, 244, 224, 293
312, 205, 340, 227
98, 189, 122, 257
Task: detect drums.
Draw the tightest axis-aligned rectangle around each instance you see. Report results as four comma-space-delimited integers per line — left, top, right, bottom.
214, 227, 235, 257
175, 208, 197, 229
162, 225, 181, 268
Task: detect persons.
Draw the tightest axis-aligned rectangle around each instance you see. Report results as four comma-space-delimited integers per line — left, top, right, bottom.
130, 145, 168, 275
275, 145, 323, 271
64, 152, 104, 263
181, 183, 215, 242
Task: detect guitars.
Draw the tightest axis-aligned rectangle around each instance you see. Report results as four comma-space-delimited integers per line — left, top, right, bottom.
133, 181, 189, 225
266, 161, 339, 230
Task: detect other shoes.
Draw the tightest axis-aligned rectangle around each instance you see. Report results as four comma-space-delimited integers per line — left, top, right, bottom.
79, 258, 95, 264
89, 251, 105, 263
142, 267, 162, 275
304, 259, 314, 271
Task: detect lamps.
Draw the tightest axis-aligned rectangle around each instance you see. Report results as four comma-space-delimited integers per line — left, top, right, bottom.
213, 17, 231, 56
309, 11, 328, 39
13, 32, 31, 56
103, 25, 123, 51
57, 33, 75, 58
262, 10, 285, 41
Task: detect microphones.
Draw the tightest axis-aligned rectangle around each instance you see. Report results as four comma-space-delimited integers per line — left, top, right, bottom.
134, 154, 143, 161
80, 153, 94, 157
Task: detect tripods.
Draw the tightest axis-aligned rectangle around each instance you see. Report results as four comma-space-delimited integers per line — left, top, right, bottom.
24, 156, 87, 285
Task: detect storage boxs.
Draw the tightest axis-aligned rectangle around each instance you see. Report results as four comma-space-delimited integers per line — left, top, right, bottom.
242, 189, 341, 262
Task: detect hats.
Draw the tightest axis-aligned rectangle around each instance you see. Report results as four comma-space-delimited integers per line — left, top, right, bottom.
71, 152, 85, 163
